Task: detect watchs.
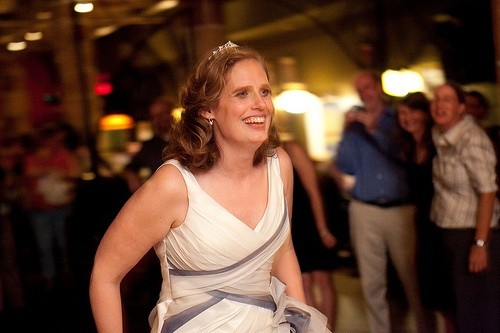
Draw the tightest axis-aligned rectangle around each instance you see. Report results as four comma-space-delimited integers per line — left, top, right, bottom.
473, 239, 486, 247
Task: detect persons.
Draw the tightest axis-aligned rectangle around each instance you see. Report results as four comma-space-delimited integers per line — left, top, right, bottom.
89, 40, 332, 332
0, 70, 500, 333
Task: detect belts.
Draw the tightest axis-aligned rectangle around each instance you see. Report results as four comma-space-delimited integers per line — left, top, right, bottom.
366, 201, 409, 208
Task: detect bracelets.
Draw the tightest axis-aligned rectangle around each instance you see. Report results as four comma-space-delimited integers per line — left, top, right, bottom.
320, 229, 328, 237
